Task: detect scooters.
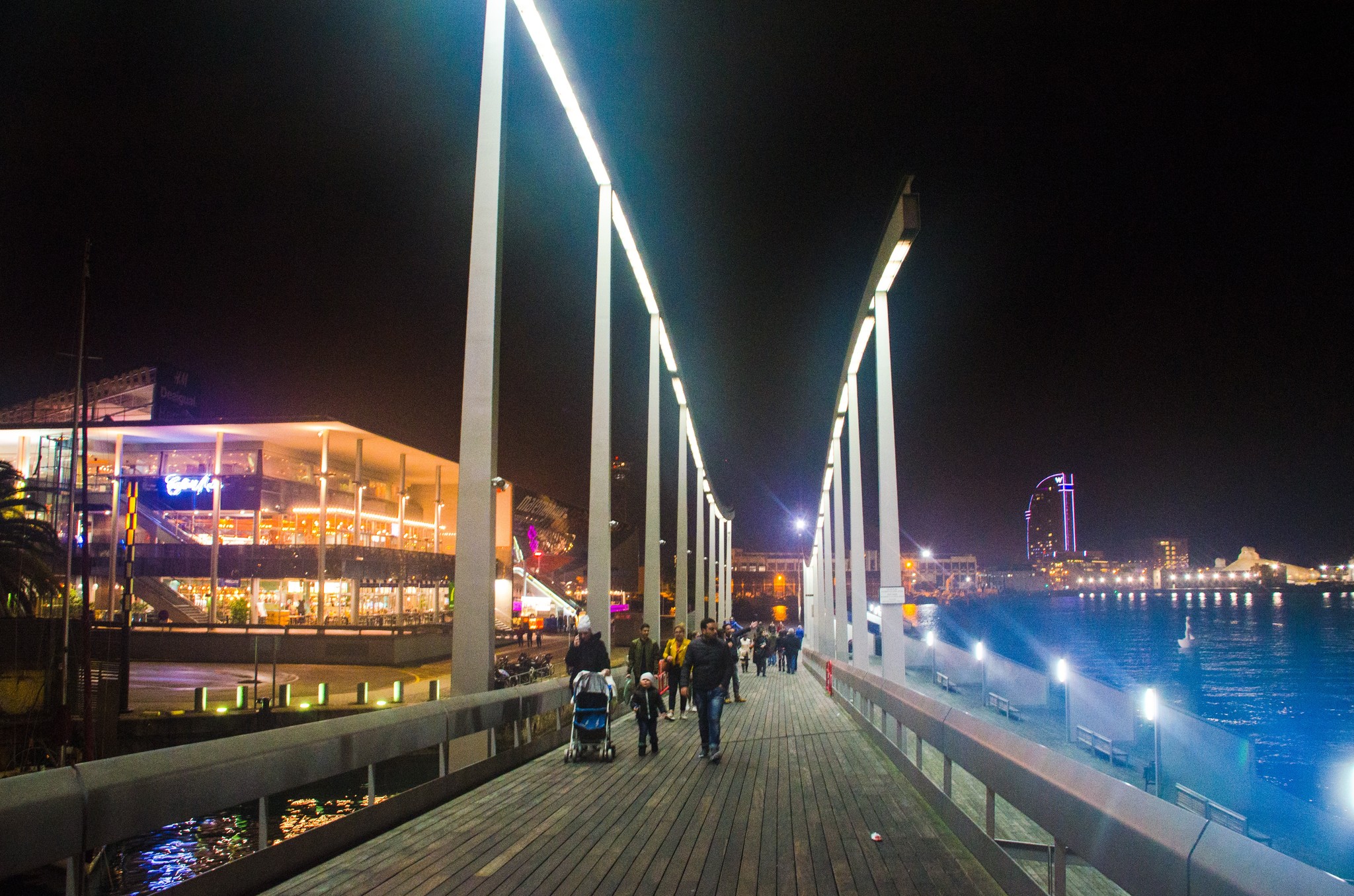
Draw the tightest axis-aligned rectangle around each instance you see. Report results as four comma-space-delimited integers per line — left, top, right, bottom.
494, 648, 554, 688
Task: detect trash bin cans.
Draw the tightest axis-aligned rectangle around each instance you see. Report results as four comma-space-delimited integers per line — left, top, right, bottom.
279, 684, 290, 707
393, 681, 403, 702
429, 680, 440, 701
237, 685, 248, 709
194, 687, 207, 711
357, 681, 368, 704
318, 683, 329, 705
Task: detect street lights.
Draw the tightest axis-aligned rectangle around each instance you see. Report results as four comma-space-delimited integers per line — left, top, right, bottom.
1058, 658, 1070, 742
1144, 688, 1159, 798
974, 640, 986, 709
926, 629, 934, 683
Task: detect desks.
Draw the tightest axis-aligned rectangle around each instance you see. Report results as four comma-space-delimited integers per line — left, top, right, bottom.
289, 610, 453, 626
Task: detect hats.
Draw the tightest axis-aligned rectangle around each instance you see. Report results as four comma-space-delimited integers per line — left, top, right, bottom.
578, 614, 591, 632
640, 672, 654, 684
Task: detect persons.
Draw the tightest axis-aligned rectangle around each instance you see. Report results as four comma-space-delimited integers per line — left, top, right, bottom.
514, 615, 804, 761
158, 606, 169, 623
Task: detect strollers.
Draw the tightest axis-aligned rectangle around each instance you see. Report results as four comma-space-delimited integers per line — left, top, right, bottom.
564, 673, 616, 764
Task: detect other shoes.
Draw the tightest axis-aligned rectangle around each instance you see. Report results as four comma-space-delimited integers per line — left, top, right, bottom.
742, 668, 745, 673
757, 671, 760, 676
794, 668, 797, 672
710, 748, 721, 760
699, 745, 709, 757
692, 705, 697, 711
638, 746, 645, 755
762, 674, 767, 677
651, 741, 659, 753
783, 669, 785, 671
779, 669, 781, 671
787, 671, 790, 673
745, 668, 748, 672
685, 704, 691, 710
791, 672, 795, 674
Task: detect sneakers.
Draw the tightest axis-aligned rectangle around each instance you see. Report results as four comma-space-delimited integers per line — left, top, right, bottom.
680, 713, 688, 720
735, 697, 746, 702
665, 712, 676, 721
724, 698, 732, 704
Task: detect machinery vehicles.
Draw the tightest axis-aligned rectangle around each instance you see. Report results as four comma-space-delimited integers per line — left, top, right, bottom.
941, 573, 955, 594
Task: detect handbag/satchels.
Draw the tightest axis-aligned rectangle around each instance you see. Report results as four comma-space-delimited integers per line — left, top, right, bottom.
623, 677, 633, 704
661, 659, 671, 672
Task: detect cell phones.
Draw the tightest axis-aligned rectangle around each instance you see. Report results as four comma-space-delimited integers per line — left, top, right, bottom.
577, 633, 580, 640
